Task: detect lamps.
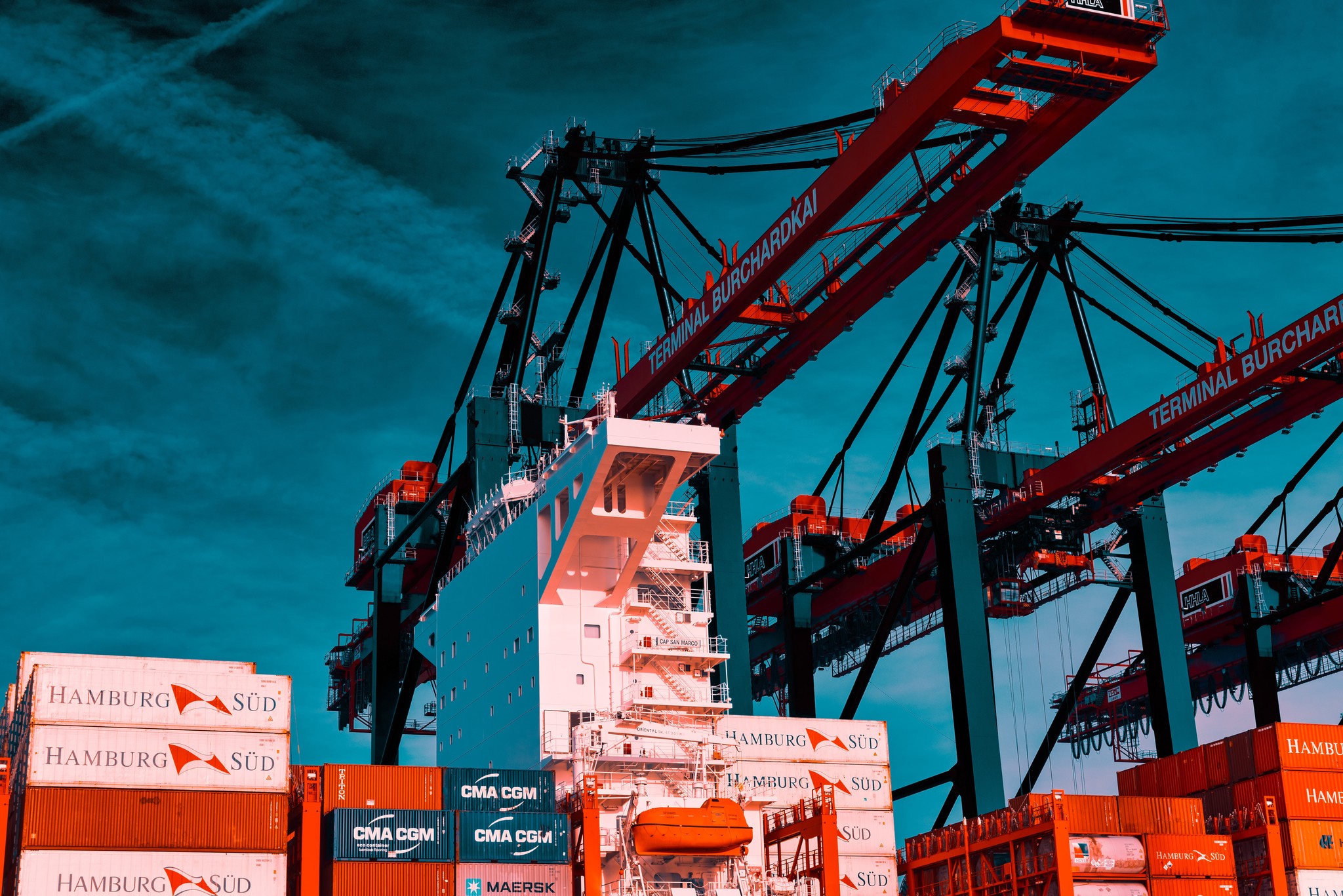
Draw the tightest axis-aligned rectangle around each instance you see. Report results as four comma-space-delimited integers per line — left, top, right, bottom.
753, 399, 763, 407
732, 416, 742, 424
1014, 176, 1026, 188
715, 863, 727, 867
634, 861, 641, 866
785, 371, 796, 380
651, 862, 663, 865
606, 807, 618, 809
843, 321, 854, 332
807, 352, 818, 361
883, 288, 895, 298
972, 211, 983, 223
926, 249, 939, 261
773, 861, 779, 868
625, 534, 715, 721
697, 413, 711, 427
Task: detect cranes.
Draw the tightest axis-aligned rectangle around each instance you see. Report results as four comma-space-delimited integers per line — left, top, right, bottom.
716, 193, 1343, 895
1048, 423, 1343, 766
321, 2, 1173, 767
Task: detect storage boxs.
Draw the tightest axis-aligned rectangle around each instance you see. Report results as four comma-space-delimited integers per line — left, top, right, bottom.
323, 764, 574, 896
573, 719, 900, 896
0, 651, 291, 896
905, 793, 1118, 861
1116, 721, 1343, 895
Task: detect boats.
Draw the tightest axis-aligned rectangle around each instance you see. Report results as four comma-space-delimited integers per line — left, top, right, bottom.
627, 794, 753, 861
